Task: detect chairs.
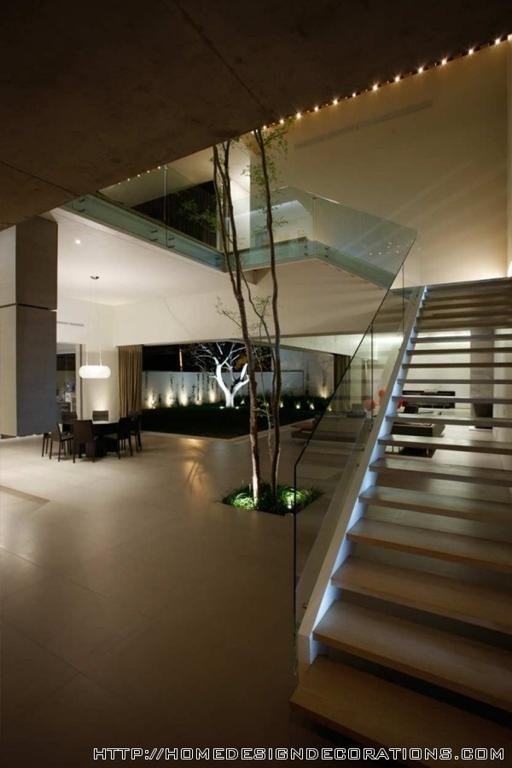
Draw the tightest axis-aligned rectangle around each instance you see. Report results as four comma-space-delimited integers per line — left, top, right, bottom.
41, 410, 143, 464
